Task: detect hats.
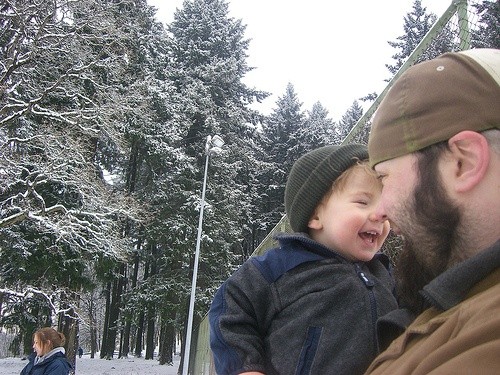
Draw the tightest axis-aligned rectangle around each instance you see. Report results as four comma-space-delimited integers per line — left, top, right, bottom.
368, 48, 500, 170
285, 144, 370, 232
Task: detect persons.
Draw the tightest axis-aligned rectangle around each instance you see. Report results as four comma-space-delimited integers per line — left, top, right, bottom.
366, 47, 500, 375
14, 327, 73, 375
209, 142, 401, 375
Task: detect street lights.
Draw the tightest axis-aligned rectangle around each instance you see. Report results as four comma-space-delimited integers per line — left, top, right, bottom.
182, 133, 225, 375
